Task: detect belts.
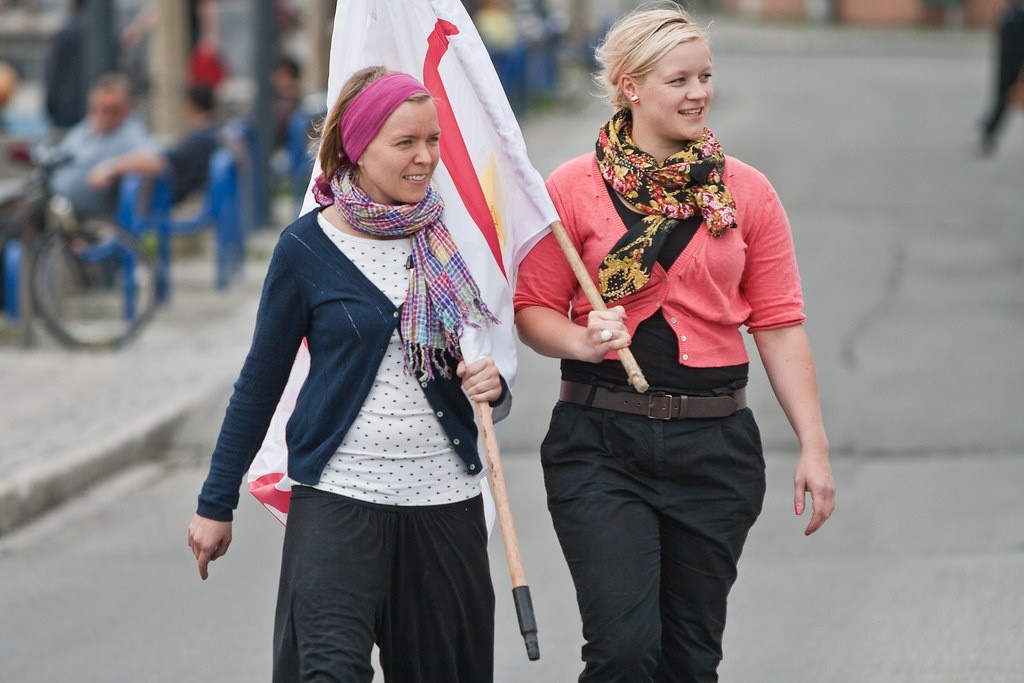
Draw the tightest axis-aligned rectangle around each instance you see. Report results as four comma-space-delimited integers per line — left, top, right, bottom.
557, 376, 748, 420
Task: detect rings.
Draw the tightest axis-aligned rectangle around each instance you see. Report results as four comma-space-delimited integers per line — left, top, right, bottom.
601, 329, 612, 341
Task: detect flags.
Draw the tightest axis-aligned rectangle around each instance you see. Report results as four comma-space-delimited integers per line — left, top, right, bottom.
245, 0, 561, 525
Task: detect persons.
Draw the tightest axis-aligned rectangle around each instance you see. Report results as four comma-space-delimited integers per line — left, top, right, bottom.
977, 0, 1023, 158
188, 66, 517, 683
514, 1, 835, 683
1, 48, 305, 350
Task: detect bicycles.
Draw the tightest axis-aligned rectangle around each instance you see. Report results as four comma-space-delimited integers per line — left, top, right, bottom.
1, 155, 164, 343
